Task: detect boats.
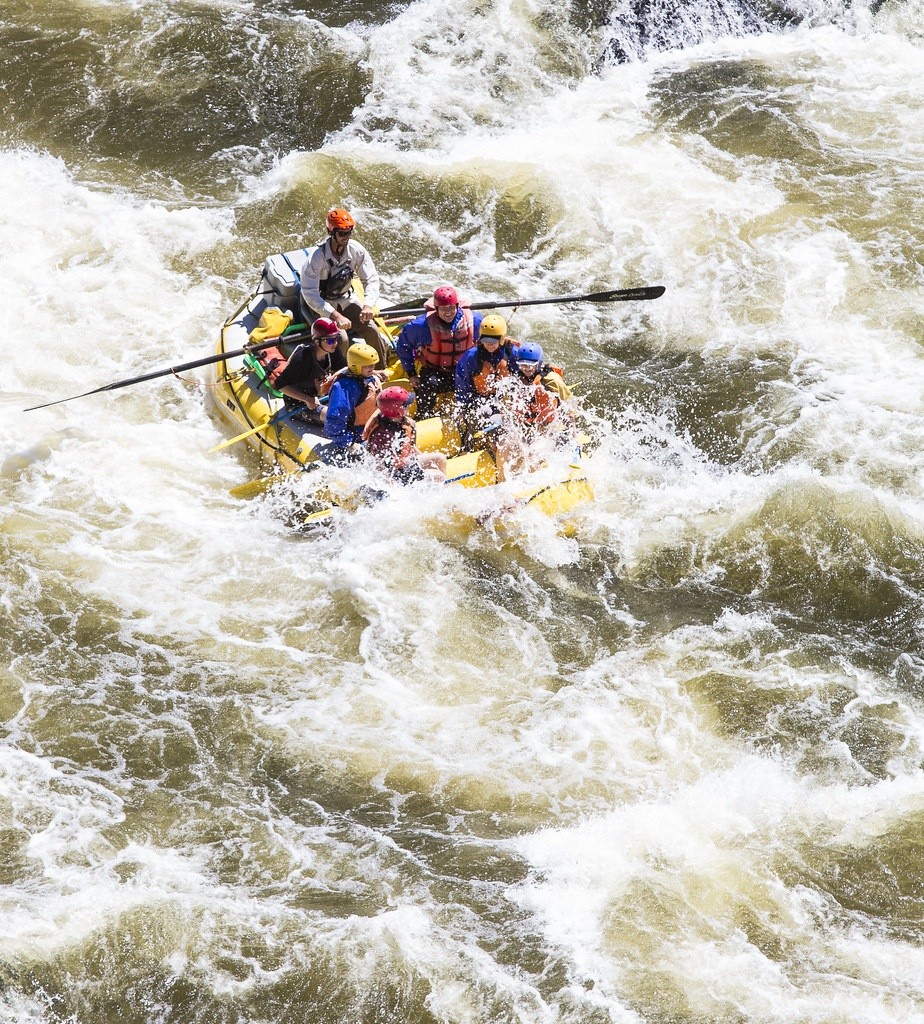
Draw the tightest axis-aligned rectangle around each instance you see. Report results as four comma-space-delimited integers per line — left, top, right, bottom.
209, 244, 597, 554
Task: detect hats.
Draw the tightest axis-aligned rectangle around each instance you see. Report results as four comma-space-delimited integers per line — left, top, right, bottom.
403, 392, 415, 407
516, 359, 538, 366
479, 335, 499, 344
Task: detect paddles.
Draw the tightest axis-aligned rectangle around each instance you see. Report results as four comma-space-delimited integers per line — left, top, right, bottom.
302, 471, 477, 525
358, 285, 667, 323
473, 381, 583, 439
206, 394, 330, 454
350, 277, 398, 352
19, 321, 342, 414
570, 434, 592, 454
227, 451, 351, 502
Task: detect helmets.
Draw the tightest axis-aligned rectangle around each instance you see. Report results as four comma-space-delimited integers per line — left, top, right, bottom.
377, 385, 415, 418
479, 315, 507, 346
347, 343, 379, 376
516, 343, 545, 372
434, 287, 459, 307
311, 317, 339, 341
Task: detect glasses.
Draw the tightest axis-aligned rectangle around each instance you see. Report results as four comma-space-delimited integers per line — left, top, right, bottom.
325, 209, 354, 235
321, 336, 339, 345
335, 229, 353, 237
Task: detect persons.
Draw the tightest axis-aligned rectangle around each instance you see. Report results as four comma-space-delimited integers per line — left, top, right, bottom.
299, 209, 381, 359
272, 287, 567, 485
